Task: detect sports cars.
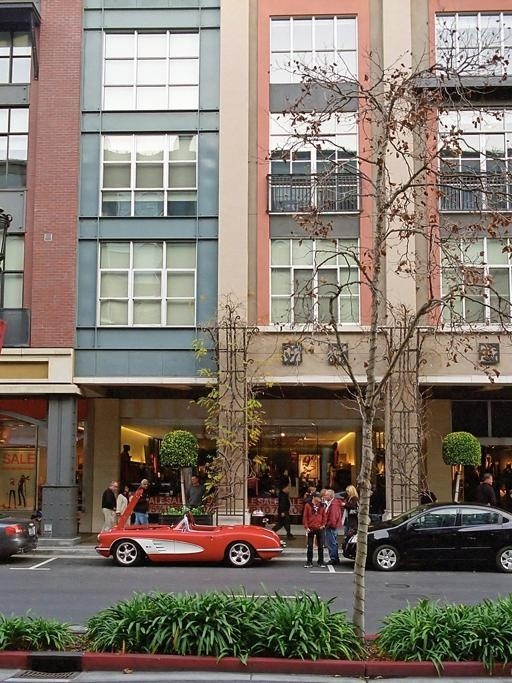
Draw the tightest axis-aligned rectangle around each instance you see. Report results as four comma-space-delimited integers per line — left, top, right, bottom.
92, 487, 284, 566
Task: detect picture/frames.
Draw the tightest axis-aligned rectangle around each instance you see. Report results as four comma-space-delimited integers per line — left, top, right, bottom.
297, 453, 322, 488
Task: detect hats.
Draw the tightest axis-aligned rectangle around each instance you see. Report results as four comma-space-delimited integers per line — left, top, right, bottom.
312, 491, 321, 497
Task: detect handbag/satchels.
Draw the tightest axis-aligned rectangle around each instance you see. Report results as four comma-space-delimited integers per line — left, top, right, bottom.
348, 495, 360, 515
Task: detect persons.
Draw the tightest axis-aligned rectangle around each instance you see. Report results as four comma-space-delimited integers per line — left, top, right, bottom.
185, 475, 205, 508
103, 478, 149, 529
8, 475, 29, 509
272, 485, 295, 540
120, 444, 132, 483
477, 473, 510, 512
302, 484, 360, 566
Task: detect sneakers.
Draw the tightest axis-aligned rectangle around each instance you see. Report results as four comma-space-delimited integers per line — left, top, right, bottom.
304, 558, 340, 568
287, 534, 296, 540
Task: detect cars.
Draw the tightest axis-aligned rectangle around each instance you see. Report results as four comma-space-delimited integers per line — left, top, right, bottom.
0, 505, 40, 562
342, 501, 512, 575
